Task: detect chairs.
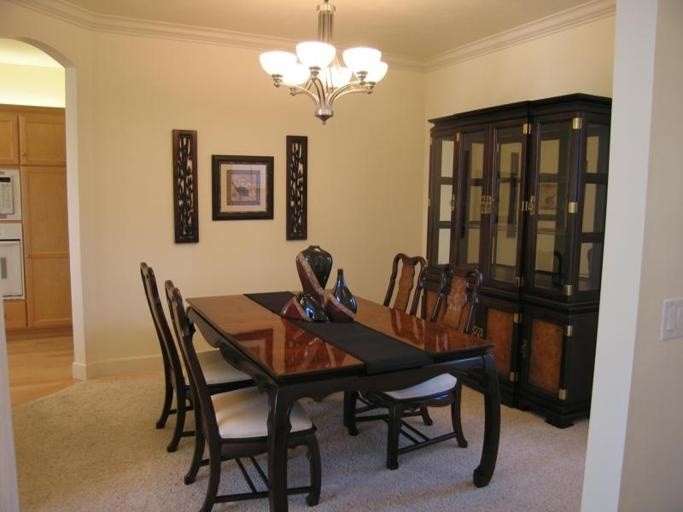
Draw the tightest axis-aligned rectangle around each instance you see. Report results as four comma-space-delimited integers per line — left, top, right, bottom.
134, 260, 256, 455
364, 260, 488, 472
336, 252, 426, 427
161, 279, 322, 511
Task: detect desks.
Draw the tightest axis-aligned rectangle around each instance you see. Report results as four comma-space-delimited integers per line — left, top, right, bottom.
182, 288, 502, 512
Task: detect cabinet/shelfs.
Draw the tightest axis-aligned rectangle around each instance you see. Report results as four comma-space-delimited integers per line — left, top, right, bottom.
422, 89, 613, 427
0, 110, 67, 167
18, 166, 73, 341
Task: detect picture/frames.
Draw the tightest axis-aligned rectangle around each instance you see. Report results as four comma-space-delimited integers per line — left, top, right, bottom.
536, 177, 564, 223
210, 154, 274, 222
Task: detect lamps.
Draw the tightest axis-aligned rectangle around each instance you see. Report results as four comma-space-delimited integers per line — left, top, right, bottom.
260, 0, 390, 125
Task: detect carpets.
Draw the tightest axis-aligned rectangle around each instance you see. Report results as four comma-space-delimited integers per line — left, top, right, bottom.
15, 379, 589, 511
